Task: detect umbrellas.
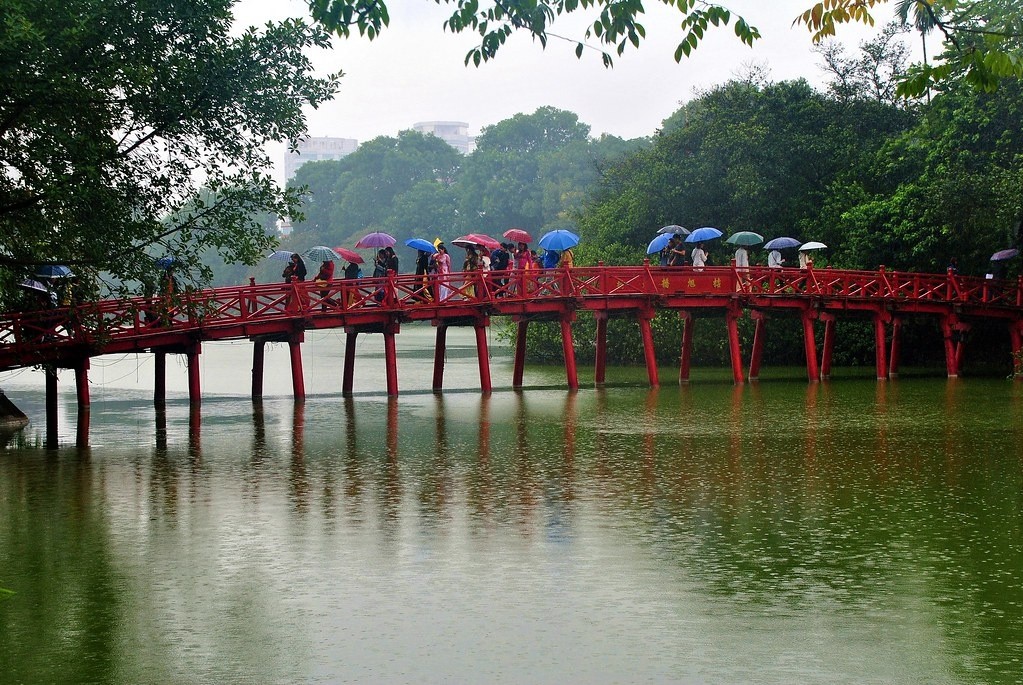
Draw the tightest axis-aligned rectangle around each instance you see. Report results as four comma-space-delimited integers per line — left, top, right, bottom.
536, 227, 580, 252
17, 265, 76, 292
725, 231, 827, 250
502, 228, 533, 243
989, 248, 1019, 260
684, 227, 724, 243
647, 224, 690, 255
451, 234, 500, 249
266, 231, 396, 264
404, 237, 438, 254
156, 257, 175, 268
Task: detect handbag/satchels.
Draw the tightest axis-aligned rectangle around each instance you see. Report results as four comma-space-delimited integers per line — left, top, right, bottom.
458, 285, 476, 298
316, 279, 327, 288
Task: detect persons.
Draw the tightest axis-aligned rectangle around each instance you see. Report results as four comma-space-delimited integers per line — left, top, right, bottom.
156, 268, 179, 328
434, 237, 450, 307
282, 247, 400, 313
533, 248, 559, 296
559, 248, 576, 297
412, 250, 438, 305
691, 241, 709, 272
734, 245, 824, 292
659, 234, 686, 272
462, 242, 544, 302
